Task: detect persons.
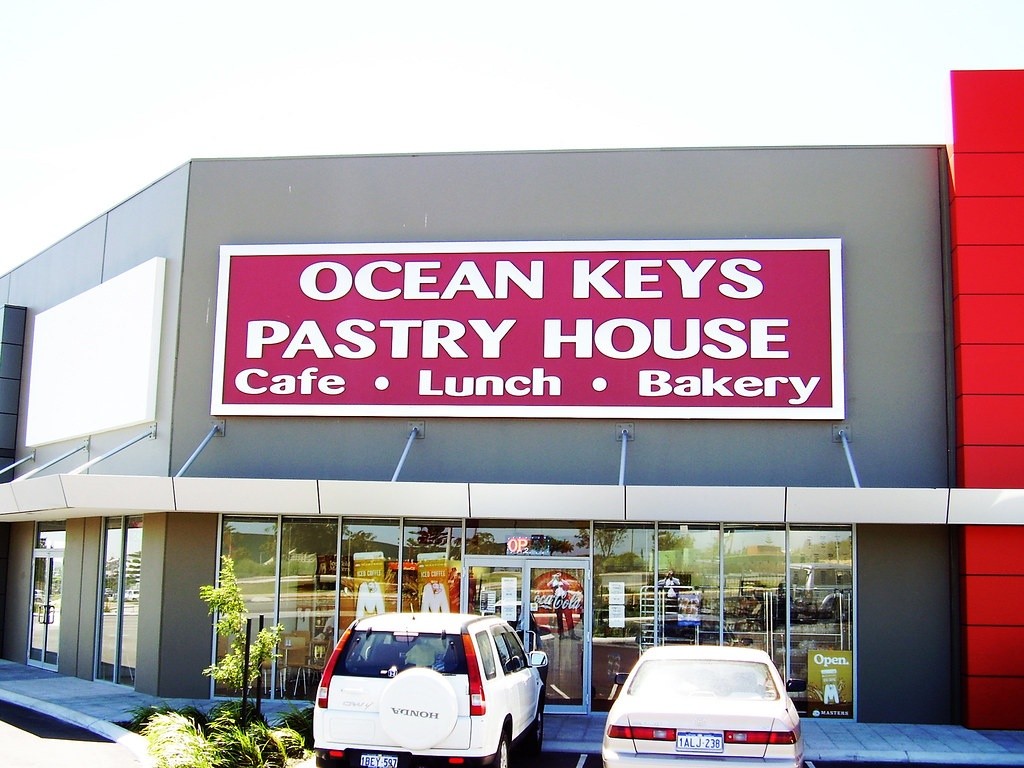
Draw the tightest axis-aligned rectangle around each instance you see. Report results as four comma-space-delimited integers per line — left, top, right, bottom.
547, 572, 581, 639
659, 571, 680, 611
448, 568, 461, 587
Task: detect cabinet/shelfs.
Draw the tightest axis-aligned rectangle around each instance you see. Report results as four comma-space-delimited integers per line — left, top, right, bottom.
695, 591, 842, 699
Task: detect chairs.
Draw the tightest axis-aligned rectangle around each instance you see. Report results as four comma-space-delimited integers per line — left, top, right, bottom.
262, 631, 334, 697
371, 644, 399, 661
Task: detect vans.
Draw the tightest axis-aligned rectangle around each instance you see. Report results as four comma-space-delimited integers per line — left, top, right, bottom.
789, 563, 853, 619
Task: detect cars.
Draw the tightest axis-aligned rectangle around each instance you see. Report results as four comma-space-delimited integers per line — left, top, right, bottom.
112, 589, 118, 599
124, 590, 139, 601
34, 590, 42, 602
602, 645, 807, 768
482, 600, 521, 617
104, 588, 113, 597
635, 614, 754, 648
577, 617, 657, 701
313, 612, 548, 768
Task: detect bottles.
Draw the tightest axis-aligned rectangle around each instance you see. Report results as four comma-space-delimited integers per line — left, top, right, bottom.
821, 669, 839, 704
692, 573, 785, 614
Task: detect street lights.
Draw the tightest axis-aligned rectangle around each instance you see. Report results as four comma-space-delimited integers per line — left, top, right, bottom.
348, 535, 354, 576
